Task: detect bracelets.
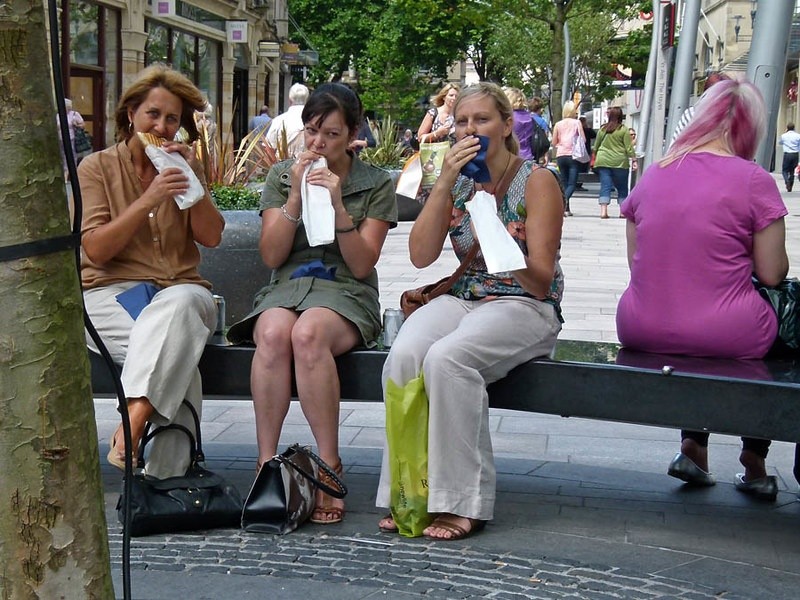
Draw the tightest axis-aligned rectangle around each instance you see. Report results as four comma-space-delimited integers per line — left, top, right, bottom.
433, 131, 436, 138
633, 160, 638, 162
335, 224, 357, 233
280, 204, 301, 223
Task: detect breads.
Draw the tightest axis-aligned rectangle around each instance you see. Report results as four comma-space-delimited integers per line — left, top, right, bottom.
136, 131, 166, 147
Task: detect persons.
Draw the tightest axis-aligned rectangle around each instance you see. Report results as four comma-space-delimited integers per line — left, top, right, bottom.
68, 62, 226, 481
664, 72, 731, 157
250, 105, 273, 144
375, 82, 567, 540
402, 82, 462, 152
56, 98, 91, 183
779, 123, 800, 192
552, 99, 587, 217
591, 107, 639, 219
619, 80, 789, 499
501, 86, 536, 163
529, 96, 549, 168
225, 82, 399, 523
347, 119, 381, 156
574, 117, 596, 191
261, 82, 310, 161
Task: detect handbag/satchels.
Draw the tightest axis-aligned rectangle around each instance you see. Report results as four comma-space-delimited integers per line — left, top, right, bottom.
400, 275, 453, 320
590, 154, 596, 168
752, 274, 800, 384
419, 135, 452, 189
385, 368, 431, 537
572, 121, 590, 163
395, 134, 432, 200
530, 112, 550, 160
116, 396, 244, 538
240, 443, 347, 535
73, 112, 92, 154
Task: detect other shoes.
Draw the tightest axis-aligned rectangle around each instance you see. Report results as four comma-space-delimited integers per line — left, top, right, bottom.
563, 212, 573, 217
786, 180, 790, 189
734, 473, 778, 504
788, 189, 792, 192
667, 452, 716, 486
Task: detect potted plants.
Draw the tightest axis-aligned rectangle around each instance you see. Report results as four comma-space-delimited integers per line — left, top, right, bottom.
358, 115, 424, 222
180, 102, 303, 325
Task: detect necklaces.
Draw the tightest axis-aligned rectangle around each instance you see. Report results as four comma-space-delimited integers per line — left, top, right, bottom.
481, 152, 511, 194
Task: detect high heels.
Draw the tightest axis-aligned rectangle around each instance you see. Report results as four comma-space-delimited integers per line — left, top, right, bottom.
107, 422, 138, 472
311, 456, 345, 524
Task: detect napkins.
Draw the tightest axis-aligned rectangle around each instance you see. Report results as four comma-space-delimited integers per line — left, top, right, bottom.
114, 283, 158, 322
289, 259, 338, 282
460, 135, 492, 185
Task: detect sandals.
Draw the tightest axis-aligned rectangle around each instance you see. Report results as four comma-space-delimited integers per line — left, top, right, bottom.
379, 515, 398, 533
424, 513, 489, 542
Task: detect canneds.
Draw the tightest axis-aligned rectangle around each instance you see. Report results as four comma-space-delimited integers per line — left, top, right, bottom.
383, 308, 404, 348
778, 277, 800, 299
212, 294, 225, 333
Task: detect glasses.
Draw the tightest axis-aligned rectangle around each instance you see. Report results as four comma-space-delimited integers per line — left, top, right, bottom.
630, 134, 636, 136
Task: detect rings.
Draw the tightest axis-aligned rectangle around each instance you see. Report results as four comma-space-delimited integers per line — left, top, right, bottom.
328, 172, 332, 176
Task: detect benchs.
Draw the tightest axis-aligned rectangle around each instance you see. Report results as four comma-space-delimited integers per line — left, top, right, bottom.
89, 339, 800, 442
